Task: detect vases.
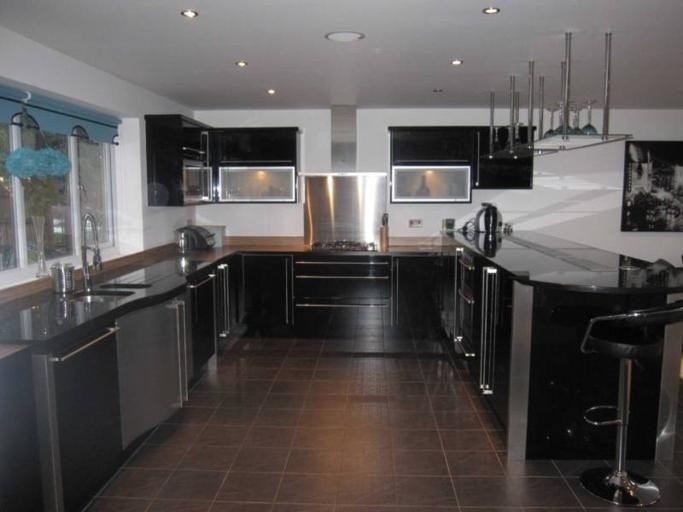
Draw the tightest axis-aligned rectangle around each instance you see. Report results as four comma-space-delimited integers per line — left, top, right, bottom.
30, 216, 52, 277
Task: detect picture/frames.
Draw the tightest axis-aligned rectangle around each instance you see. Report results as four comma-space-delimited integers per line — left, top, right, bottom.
619, 139, 682, 231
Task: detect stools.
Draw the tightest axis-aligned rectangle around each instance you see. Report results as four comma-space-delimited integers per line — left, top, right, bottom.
578, 299, 683, 508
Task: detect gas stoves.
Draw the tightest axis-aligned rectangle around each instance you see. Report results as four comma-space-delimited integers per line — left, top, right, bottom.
311, 239, 375, 251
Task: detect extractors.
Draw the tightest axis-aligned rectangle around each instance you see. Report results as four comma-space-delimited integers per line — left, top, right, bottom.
297, 105, 390, 178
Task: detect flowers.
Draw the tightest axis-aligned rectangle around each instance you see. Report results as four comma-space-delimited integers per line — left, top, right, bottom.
7, 145, 73, 259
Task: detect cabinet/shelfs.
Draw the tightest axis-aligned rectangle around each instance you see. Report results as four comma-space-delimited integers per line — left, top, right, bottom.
213, 127, 301, 165
233, 253, 294, 351
31, 322, 124, 511
455, 247, 479, 384
143, 115, 212, 207
188, 253, 243, 394
293, 255, 393, 352
473, 125, 537, 190
387, 126, 477, 164
477, 256, 667, 468
391, 258, 460, 355
115, 291, 196, 462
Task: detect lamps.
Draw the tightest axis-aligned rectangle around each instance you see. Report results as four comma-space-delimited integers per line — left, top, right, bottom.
479, 30, 635, 164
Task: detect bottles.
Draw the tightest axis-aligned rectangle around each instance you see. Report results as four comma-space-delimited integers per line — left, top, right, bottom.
414, 173, 431, 196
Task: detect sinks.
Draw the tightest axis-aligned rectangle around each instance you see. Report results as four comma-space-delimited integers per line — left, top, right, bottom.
71, 291, 134, 301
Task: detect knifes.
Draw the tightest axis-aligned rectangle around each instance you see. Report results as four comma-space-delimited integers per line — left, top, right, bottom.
381, 212, 389, 227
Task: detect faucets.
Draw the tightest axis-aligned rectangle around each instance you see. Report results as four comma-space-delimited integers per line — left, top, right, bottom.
79, 214, 104, 289
